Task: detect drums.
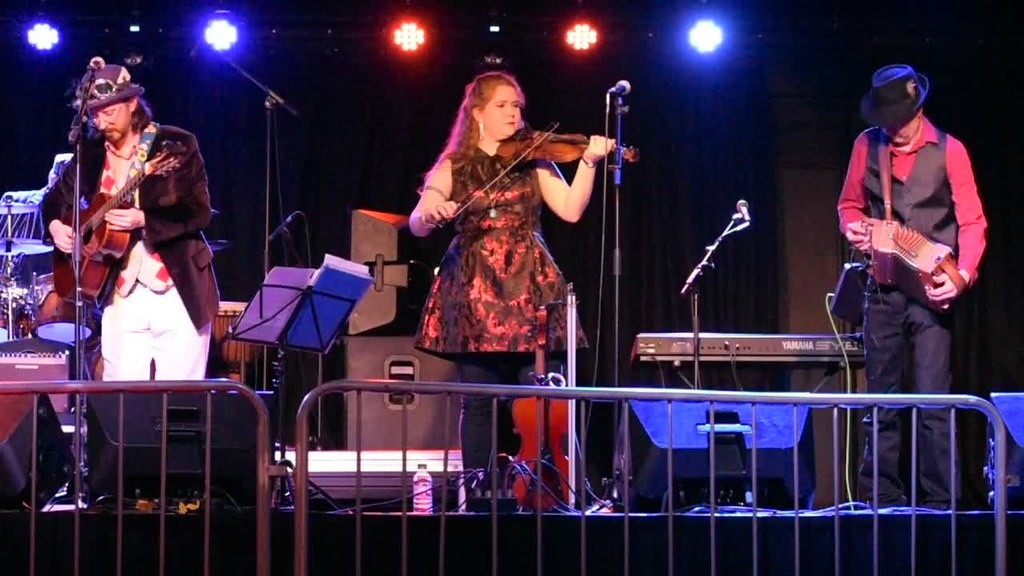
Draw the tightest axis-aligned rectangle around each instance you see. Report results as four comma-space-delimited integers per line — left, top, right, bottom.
31, 271, 94, 344
0, 274, 32, 337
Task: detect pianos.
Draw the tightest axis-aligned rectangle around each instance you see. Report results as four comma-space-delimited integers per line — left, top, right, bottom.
629, 331, 865, 505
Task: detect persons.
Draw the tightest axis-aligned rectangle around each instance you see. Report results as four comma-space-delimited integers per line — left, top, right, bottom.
836, 60, 987, 508
36, 64, 220, 505
410, 72, 616, 509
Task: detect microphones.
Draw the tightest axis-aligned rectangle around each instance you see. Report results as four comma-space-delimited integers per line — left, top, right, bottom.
608, 80, 632, 95
89, 56, 106, 71
268, 211, 301, 241
190, 37, 205, 57
736, 199, 751, 221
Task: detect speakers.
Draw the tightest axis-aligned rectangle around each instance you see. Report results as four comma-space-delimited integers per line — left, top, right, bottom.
340, 217, 464, 449
612, 398, 816, 513
0, 393, 75, 509
985, 392, 1024, 504
88, 390, 278, 504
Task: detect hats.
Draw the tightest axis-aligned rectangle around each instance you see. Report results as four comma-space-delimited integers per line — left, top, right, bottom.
860, 64, 930, 129
74, 64, 145, 114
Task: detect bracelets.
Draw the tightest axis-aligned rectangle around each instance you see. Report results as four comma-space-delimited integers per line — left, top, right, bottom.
422, 215, 435, 228
581, 156, 596, 168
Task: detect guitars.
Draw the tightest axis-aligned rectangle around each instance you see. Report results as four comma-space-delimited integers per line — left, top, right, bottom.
54, 141, 186, 305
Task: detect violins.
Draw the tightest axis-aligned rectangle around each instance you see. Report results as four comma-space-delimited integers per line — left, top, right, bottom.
509, 304, 574, 513
497, 129, 639, 164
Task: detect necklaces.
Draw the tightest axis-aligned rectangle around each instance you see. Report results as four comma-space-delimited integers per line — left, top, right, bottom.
474, 165, 502, 217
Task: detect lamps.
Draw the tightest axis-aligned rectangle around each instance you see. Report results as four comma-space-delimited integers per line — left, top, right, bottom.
203, 8, 242, 55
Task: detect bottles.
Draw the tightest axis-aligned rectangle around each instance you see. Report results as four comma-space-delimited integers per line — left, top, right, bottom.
413, 464, 433, 514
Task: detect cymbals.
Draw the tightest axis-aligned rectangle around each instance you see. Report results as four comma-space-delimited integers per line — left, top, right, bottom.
0, 202, 40, 214
0, 237, 55, 255
